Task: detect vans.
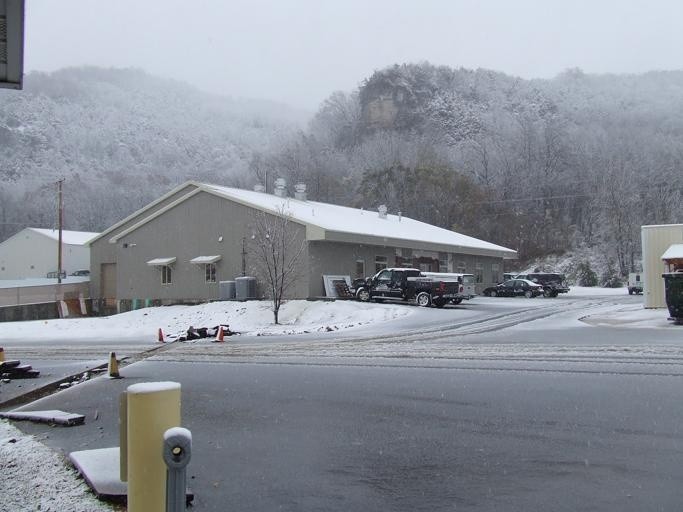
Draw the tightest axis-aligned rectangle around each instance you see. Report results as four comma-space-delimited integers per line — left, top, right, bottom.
627, 273, 643, 294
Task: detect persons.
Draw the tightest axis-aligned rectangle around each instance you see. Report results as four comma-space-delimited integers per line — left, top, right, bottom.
153, 296, 161, 306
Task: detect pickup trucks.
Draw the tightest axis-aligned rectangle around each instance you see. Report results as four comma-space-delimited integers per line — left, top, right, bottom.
351, 268, 477, 307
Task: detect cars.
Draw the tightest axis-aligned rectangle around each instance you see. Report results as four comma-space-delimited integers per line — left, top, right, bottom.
483, 273, 570, 298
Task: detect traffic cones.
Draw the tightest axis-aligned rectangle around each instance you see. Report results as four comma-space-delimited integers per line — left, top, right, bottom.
107, 352, 124, 379
214, 326, 225, 342
0, 347, 5, 364
159, 328, 163, 341
